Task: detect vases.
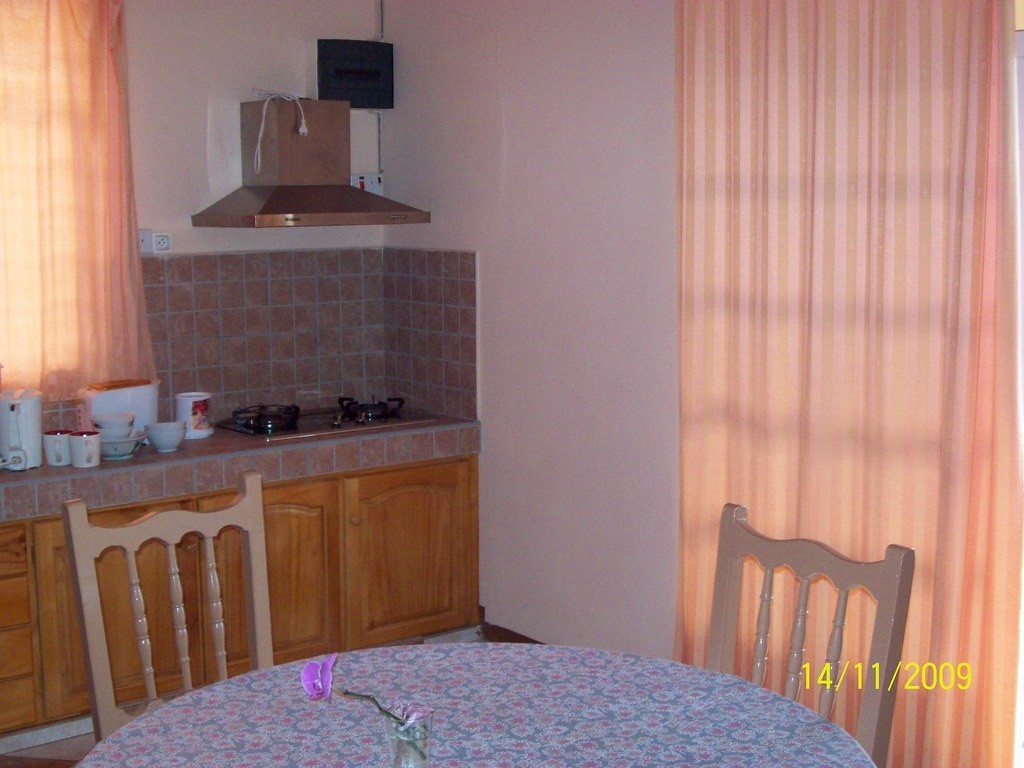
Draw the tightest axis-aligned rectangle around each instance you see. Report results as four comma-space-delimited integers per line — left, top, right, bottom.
385, 703, 434, 768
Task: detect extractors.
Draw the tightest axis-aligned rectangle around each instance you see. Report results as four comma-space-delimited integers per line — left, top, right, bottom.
191, 97, 437, 227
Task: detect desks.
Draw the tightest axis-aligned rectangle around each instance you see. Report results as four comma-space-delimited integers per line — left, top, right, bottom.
71, 641, 878, 768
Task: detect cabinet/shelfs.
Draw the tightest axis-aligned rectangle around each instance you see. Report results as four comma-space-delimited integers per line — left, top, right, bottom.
338, 454, 480, 651
31, 472, 339, 725
0, 517, 36, 734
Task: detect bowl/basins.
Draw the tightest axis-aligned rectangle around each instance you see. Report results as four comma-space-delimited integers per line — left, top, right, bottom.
144, 421, 188, 453
90, 412, 146, 460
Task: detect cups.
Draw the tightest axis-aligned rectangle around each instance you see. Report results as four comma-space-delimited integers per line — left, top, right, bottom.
69, 431, 101, 468
42, 429, 74, 466
175, 392, 215, 440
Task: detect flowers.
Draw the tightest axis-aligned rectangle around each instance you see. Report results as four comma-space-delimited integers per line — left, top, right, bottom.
301, 653, 427, 759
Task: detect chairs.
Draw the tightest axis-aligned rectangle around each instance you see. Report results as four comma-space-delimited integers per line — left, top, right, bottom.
62, 472, 274, 744
706, 502, 915, 768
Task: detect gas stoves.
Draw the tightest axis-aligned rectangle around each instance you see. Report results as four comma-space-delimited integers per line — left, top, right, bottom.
215, 398, 435, 438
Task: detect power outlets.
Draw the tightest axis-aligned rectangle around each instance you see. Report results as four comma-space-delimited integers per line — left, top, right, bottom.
154, 235, 170, 250
138, 229, 153, 253
